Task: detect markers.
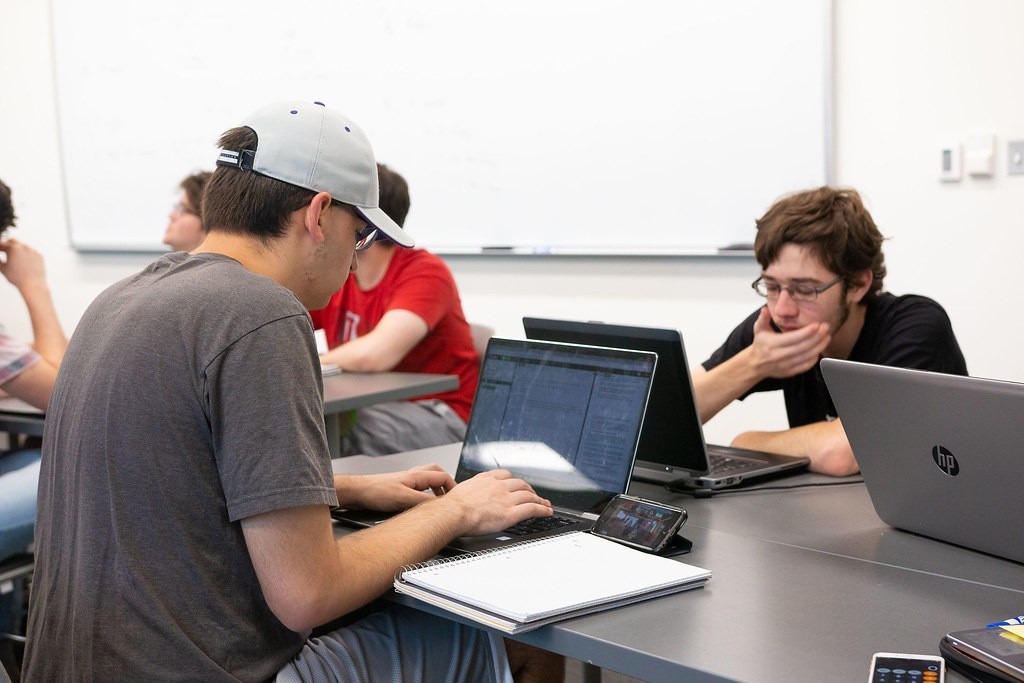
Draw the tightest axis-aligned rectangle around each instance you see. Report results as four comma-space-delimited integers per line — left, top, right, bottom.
482, 246, 552, 252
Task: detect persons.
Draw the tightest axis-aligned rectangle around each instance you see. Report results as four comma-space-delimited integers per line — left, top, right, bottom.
0, 180, 71, 564
689, 186, 970, 479
308, 161, 482, 455
18, 99, 555, 682
160, 169, 216, 253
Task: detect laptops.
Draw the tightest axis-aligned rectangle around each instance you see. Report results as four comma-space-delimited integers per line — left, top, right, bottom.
946, 624, 1024, 681
819, 357, 1024, 565
524, 316, 811, 490
333, 336, 658, 554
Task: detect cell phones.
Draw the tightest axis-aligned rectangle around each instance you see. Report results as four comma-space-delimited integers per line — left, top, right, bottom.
591, 495, 690, 551
867, 652, 946, 683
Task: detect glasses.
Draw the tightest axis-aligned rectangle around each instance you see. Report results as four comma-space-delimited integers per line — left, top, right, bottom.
331, 199, 379, 252
753, 274, 848, 303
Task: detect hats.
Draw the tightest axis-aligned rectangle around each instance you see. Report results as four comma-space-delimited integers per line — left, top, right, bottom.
216, 101, 415, 249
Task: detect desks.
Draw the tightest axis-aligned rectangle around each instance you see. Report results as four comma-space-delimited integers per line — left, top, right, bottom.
330, 440, 1024, 683
0, 370, 459, 460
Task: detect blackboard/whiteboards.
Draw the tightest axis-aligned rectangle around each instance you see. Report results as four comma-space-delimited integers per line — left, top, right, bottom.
47, 0, 837, 257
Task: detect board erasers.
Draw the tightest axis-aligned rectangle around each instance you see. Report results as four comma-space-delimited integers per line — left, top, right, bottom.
718, 243, 758, 257
